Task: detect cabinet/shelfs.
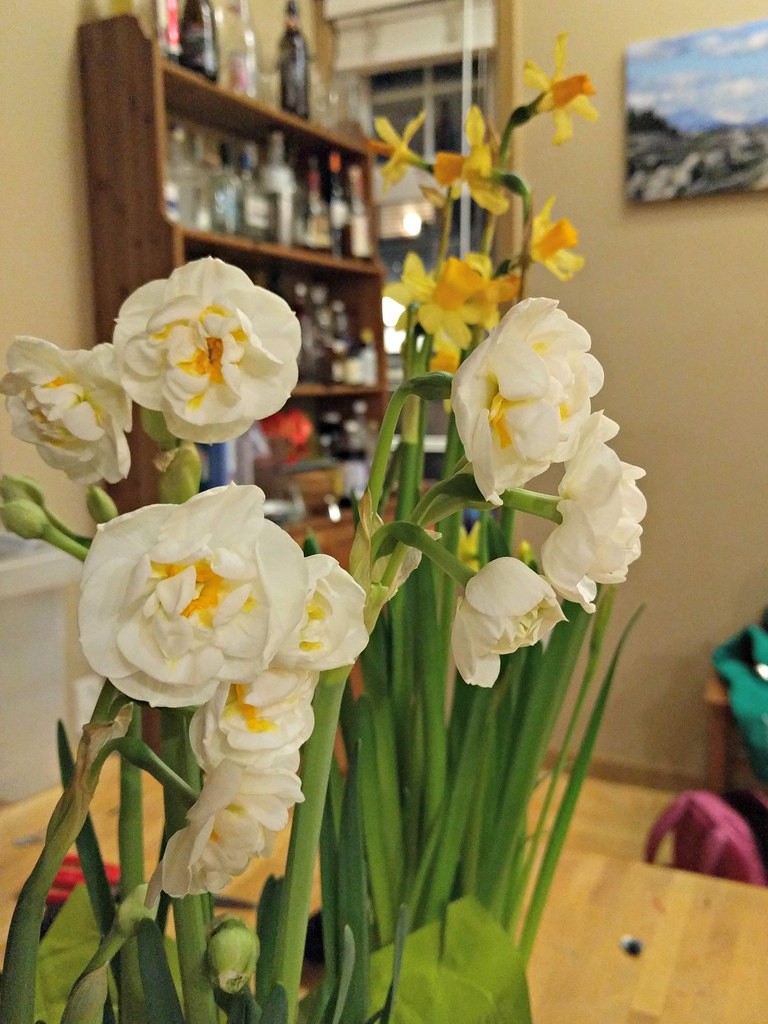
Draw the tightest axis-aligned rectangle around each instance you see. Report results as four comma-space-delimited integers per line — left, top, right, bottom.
76, 12, 437, 777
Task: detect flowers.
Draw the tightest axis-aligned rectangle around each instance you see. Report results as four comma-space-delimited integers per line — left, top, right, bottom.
0, 33, 649, 1024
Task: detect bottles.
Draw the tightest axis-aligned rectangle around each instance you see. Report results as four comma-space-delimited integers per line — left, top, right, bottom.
150, 0, 382, 517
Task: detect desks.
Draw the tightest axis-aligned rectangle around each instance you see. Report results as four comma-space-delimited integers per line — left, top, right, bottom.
0, 754, 768, 1024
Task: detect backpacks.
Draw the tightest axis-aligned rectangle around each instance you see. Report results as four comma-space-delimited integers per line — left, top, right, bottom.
645, 790, 768, 887
